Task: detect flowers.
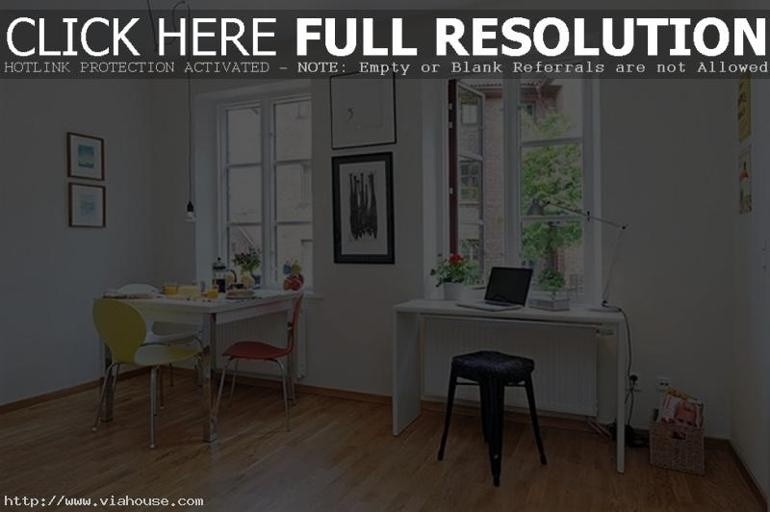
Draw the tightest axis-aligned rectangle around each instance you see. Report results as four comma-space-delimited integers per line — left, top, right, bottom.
232, 244, 262, 273
429, 251, 483, 288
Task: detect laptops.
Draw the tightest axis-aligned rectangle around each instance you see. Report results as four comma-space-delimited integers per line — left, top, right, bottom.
455, 265, 534, 312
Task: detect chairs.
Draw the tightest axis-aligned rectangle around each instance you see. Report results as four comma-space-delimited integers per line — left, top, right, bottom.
90, 296, 203, 450
113, 280, 175, 409
211, 291, 306, 420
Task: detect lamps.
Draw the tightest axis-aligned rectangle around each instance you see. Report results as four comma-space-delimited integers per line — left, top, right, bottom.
182, 202, 197, 223
526, 197, 630, 313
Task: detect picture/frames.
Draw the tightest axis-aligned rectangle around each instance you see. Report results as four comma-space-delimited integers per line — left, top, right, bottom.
65, 130, 106, 183
69, 182, 108, 230
329, 66, 398, 151
332, 150, 396, 265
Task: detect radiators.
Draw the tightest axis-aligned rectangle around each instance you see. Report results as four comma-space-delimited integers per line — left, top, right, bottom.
214, 293, 307, 381
422, 314, 614, 418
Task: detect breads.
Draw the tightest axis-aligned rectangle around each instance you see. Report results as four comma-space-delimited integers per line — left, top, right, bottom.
227, 289, 255, 298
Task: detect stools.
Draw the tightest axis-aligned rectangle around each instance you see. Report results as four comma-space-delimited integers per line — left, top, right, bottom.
436, 350, 548, 486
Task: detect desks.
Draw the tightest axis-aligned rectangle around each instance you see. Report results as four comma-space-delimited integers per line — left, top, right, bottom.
391, 299, 627, 475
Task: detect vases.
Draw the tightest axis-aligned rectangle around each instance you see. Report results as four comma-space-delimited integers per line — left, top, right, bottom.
241, 272, 255, 289
442, 281, 465, 298
